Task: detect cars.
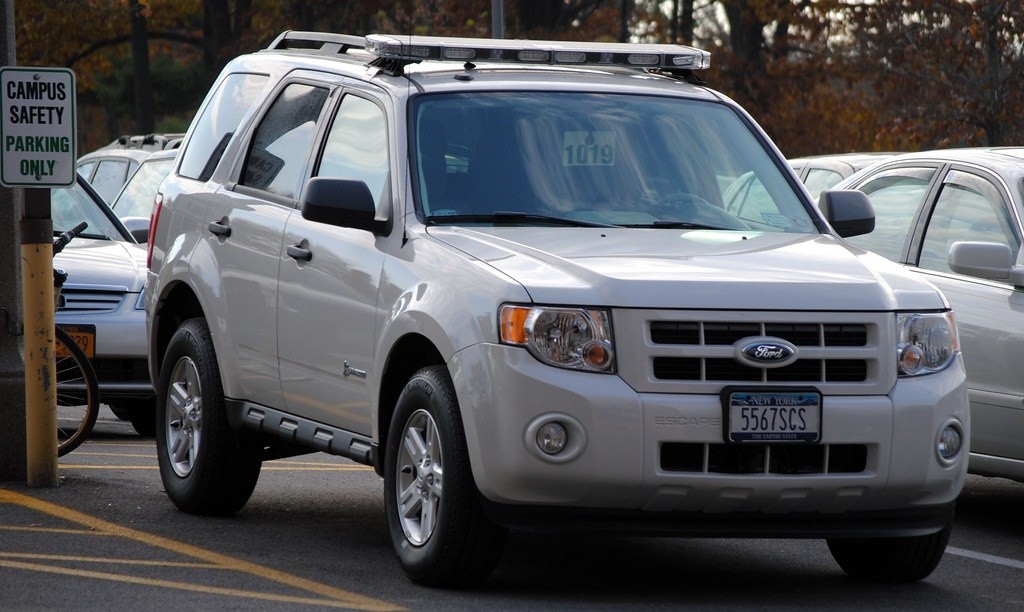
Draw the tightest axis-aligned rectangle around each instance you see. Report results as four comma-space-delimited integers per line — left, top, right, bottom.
55, 132, 192, 431
805, 146, 1024, 481
720, 153, 928, 271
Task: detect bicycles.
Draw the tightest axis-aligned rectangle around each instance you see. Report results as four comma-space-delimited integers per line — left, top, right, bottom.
53, 223, 99, 463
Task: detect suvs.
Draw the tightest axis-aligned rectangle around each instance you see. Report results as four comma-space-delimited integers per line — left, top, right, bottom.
142, 29, 970, 588
